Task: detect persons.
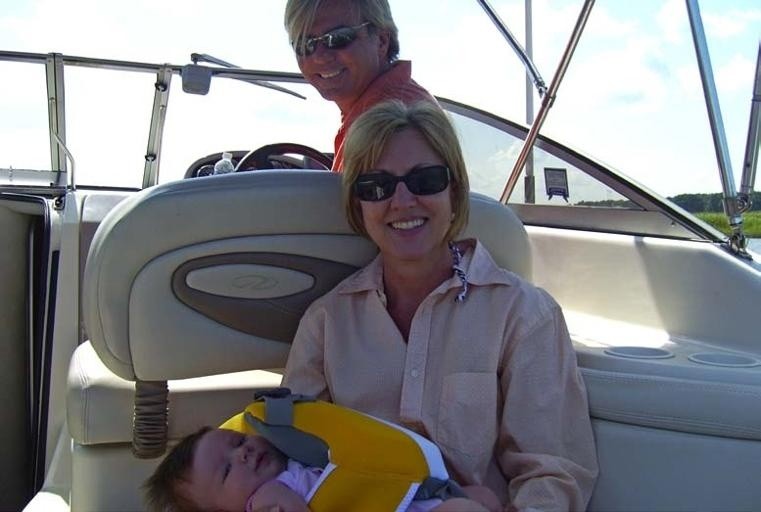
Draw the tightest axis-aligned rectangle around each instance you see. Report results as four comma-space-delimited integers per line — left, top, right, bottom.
278, 96, 601, 512
284, 1, 442, 170
140, 415, 504, 512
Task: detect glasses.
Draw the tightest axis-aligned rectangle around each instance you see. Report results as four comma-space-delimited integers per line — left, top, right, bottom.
291, 20, 372, 56
356, 165, 453, 202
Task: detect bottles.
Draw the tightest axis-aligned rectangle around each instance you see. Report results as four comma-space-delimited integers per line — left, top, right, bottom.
213, 152, 236, 176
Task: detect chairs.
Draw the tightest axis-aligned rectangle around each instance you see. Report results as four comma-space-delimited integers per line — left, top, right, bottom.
65, 169, 532, 512
